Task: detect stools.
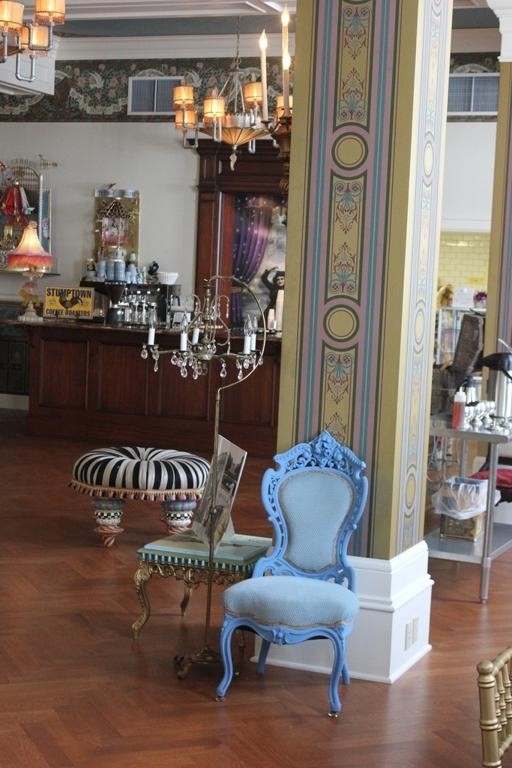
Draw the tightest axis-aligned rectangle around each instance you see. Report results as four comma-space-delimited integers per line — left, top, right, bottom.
68, 446, 212, 546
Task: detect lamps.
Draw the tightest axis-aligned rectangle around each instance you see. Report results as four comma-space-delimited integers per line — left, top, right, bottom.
0, 0, 67, 81
172, 0, 295, 171
7, 224, 53, 322
140, 277, 266, 680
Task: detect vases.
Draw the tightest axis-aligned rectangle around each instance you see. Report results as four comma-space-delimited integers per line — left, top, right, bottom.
473, 299, 486, 309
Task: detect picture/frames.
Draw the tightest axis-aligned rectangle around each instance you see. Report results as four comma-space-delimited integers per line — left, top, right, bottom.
0, 185, 53, 257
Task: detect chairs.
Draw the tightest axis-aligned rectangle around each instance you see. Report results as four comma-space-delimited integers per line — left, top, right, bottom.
476, 645, 512, 768
216, 428, 370, 719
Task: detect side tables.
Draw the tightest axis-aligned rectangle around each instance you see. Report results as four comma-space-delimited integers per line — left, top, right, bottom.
132, 529, 271, 641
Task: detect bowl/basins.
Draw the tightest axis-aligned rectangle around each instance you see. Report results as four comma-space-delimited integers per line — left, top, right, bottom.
158, 271, 178, 285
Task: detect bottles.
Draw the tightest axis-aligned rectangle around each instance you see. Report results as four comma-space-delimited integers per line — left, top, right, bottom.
452, 386, 466, 428
128, 249, 136, 284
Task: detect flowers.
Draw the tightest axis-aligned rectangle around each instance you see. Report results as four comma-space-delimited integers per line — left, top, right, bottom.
475, 291, 486, 308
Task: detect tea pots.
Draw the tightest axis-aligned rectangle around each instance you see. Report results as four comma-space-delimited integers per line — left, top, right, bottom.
481, 352, 512, 418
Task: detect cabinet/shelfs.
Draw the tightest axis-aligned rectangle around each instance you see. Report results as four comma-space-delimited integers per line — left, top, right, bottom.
424, 410, 512, 604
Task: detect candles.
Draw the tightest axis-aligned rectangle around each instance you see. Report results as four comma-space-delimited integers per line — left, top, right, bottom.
281, 52, 289, 113
281, 4, 291, 68
259, 25, 268, 119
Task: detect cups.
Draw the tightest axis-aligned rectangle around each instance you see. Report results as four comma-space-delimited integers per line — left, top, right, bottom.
98, 258, 125, 281
126, 272, 131, 284
136, 266, 146, 284
119, 288, 161, 326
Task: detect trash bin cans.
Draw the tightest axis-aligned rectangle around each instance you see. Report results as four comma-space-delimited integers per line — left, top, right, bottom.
436, 476, 488, 544
